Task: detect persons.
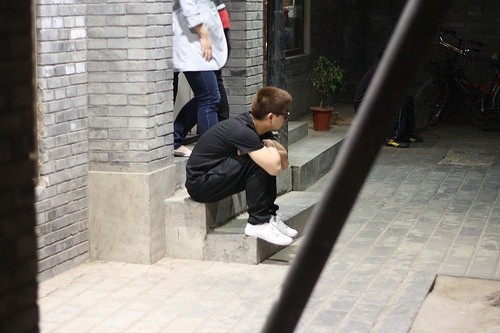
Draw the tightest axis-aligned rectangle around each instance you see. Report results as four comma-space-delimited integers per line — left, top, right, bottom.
173, 0, 228, 156
173, 0, 230, 122
354, 48, 417, 148
185, 86, 298, 246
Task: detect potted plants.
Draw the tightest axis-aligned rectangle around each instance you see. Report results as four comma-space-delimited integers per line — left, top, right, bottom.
309, 56, 343, 131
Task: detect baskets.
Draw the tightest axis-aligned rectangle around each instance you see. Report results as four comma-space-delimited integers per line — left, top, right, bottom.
426, 47, 465, 78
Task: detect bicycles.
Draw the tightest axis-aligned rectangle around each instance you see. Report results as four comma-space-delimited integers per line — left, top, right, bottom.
409, 28, 500, 130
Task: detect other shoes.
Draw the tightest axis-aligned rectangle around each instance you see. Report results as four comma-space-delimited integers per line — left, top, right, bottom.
399, 134, 423, 142
270, 214, 298, 236
385, 136, 409, 147
174, 144, 192, 156
245, 222, 292, 245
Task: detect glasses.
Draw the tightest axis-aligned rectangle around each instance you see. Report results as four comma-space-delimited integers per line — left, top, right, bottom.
275, 111, 290, 119
